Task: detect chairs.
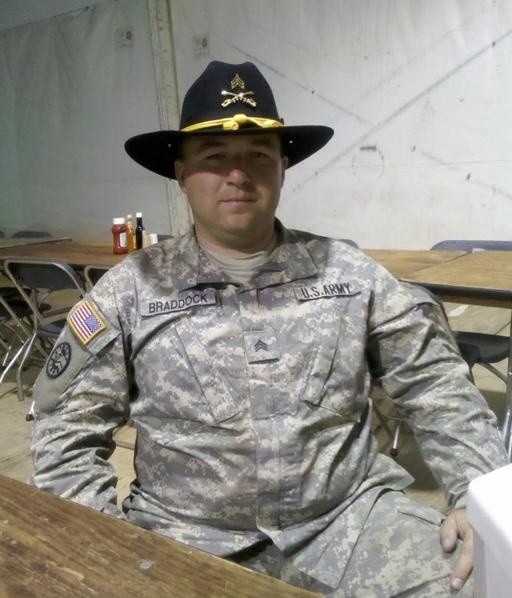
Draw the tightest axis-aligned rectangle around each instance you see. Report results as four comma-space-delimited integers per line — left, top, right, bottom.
369, 240, 512, 459
0, 258, 117, 402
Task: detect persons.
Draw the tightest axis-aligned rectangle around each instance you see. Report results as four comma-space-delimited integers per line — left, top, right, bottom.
26, 61, 511, 597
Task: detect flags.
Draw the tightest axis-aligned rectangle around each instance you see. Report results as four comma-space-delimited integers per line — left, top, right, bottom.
69, 302, 101, 340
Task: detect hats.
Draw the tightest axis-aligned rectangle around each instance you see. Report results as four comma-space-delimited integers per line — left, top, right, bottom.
124, 60, 334, 180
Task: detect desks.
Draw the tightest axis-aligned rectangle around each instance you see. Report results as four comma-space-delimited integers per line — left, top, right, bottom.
358, 250, 511, 309
0, 237, 124, 267
0, 237, 511, 403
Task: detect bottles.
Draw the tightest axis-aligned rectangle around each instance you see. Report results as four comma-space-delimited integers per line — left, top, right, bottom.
111, 217, 128, 254
126, 214, 137, 254
135, 212, 146, 249
